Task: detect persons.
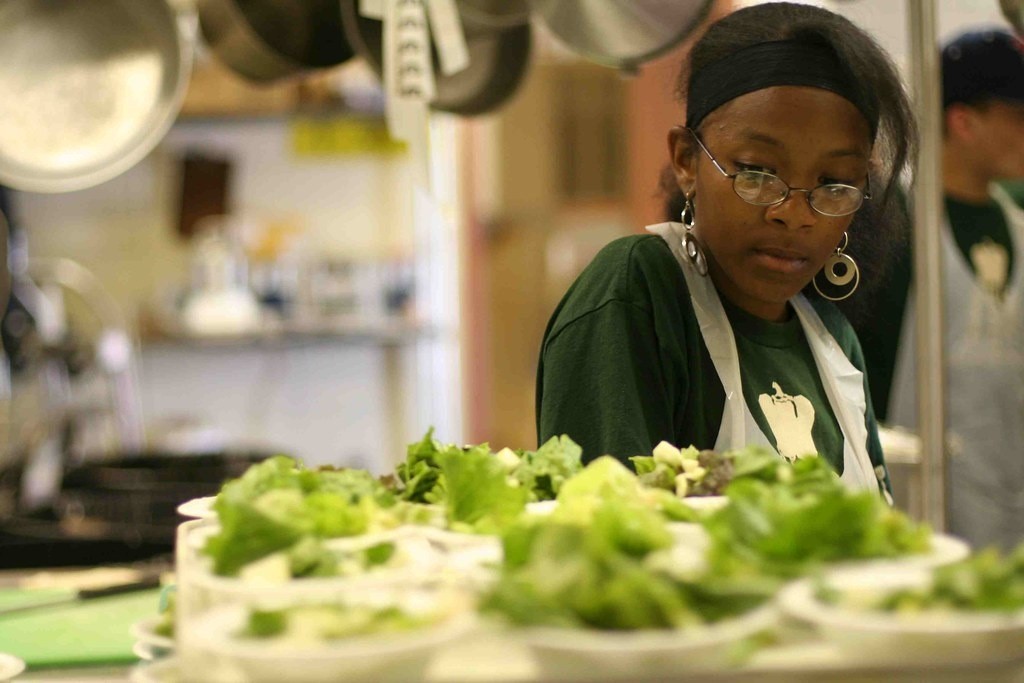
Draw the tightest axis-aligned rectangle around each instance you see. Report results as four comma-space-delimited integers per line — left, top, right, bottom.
855, 28, 1024, 554
535, 0, 912, 508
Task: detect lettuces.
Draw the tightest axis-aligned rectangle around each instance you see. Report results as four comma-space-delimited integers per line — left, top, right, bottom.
197, 429, 1024, 663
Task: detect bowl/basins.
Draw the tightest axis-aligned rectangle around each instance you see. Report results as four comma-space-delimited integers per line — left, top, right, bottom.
197, 0, 716, 119
82, 451, 275, 524
125, 493, 1024, 683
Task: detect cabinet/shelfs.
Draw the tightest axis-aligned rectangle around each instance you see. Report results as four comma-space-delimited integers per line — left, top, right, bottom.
135, 97, 437, 347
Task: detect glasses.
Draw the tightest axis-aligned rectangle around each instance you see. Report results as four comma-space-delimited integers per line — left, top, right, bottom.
689, 127, 873, 218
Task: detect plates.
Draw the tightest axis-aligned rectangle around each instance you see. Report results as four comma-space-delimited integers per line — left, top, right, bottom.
0, 0, 192, 195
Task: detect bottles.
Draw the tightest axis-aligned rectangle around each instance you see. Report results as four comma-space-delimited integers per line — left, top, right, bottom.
183, 220, 261, 336
